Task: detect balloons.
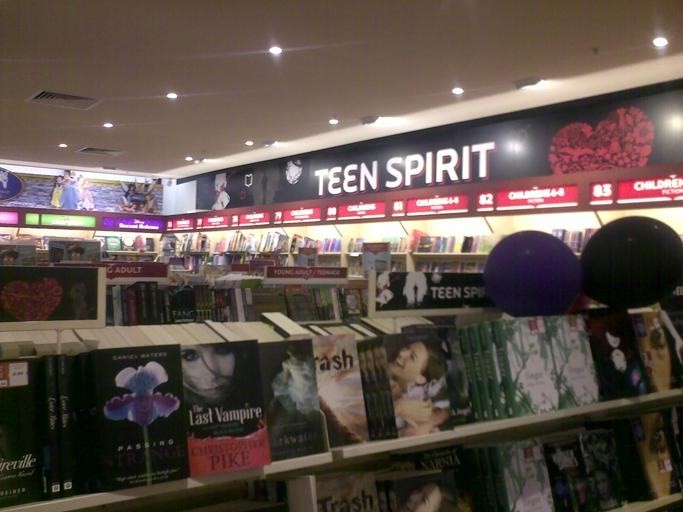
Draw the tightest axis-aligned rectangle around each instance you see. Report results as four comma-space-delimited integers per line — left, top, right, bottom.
579, 214, 682, 310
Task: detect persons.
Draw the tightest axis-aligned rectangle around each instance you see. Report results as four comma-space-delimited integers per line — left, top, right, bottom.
50, 169, 162, 216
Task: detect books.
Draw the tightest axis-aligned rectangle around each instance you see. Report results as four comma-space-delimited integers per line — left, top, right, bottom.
0, 228, 682, 512
